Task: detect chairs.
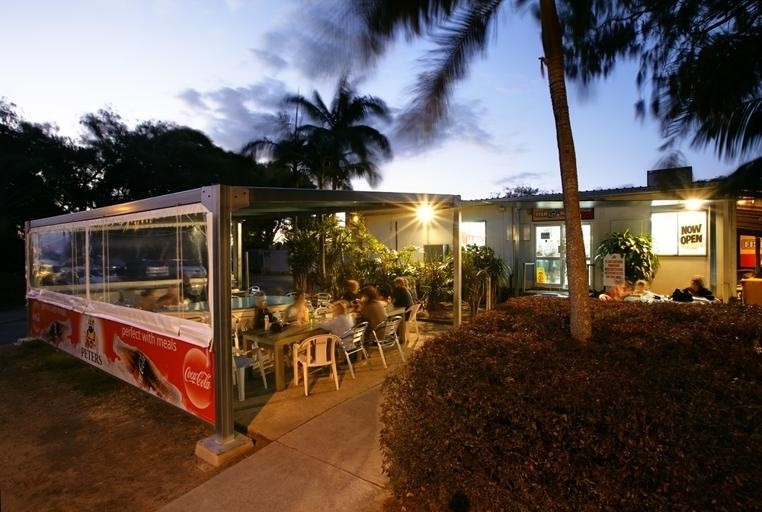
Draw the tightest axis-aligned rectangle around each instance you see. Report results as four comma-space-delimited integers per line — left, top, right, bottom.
159, 292, 422, 402
599, 294, 612, 300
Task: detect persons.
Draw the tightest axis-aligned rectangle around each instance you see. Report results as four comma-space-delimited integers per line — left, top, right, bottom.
180, 275, 201, 303
238, 290, 290, 367
156, 284, 183, 309
401, 276, 414, 295
316, 301, 354, 345
359, 286, 389, 346
606, 273, 647, 302
343, 279, 360, 304
283, 290, 308, 327
682, 275, 713, 298
139, 287, 161, 313
390, 278, 413, 336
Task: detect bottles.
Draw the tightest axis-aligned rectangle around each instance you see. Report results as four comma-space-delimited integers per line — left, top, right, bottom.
37, 319, 73, 348
264, 315, 269, 331
83, 318, 98, 354
112, 334, 183, 408
308, 311, 316, 332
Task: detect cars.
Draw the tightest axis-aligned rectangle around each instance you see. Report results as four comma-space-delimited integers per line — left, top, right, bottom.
32, 256, 207, 285
